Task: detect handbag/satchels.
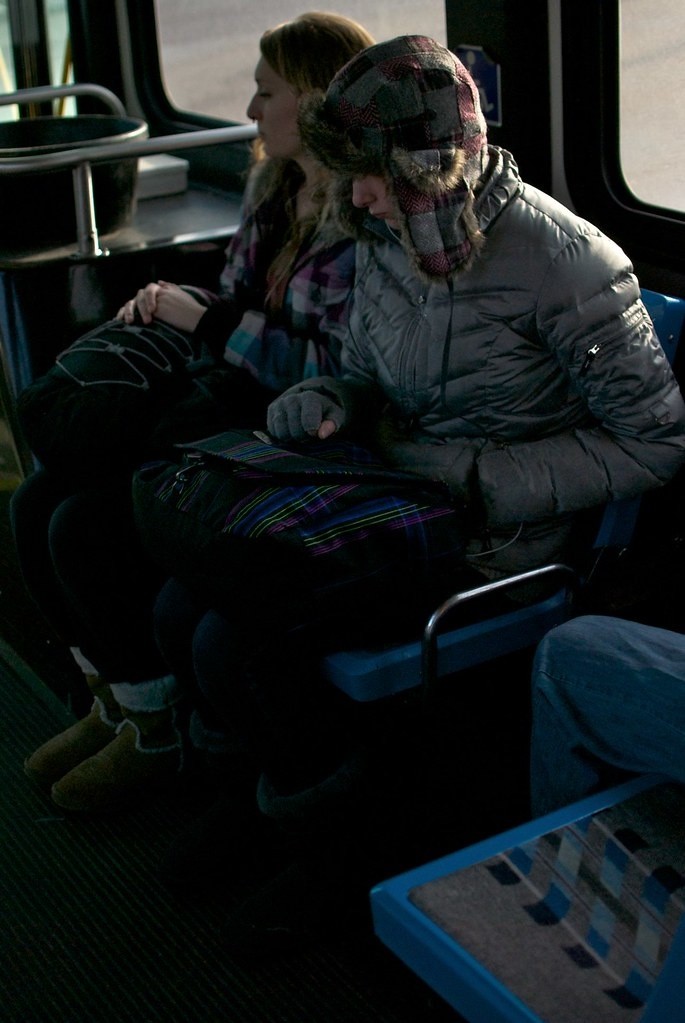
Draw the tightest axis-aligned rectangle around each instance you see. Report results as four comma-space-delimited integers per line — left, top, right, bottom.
16, 287, 241, 527
133, 431, 489, 611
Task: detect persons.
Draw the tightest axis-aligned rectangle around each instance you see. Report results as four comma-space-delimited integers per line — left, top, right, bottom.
151, 35, 685, 947
529, 614, 685, 822
9, 16, 371, 810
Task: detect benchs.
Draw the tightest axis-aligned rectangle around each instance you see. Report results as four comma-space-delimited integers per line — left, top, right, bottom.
370, 771, 685, 1023
325, 288, 685, 701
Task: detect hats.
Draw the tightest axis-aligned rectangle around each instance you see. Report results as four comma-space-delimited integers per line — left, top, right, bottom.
294, 34, 488, 285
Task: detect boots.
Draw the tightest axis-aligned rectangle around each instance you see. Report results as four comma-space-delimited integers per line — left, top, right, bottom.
165, 711, 285, 907
23, 646, 111, 788
219, 763, 355, 963
51, 669, 186, 812
